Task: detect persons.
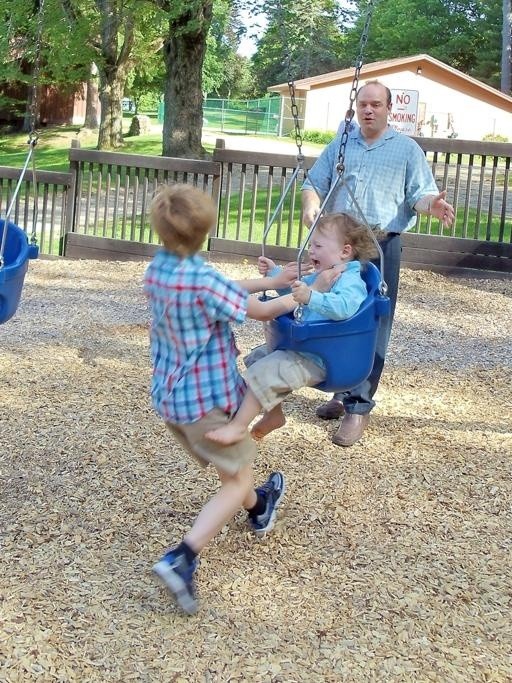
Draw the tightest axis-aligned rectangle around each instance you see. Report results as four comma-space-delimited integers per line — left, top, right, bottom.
203, 211, 387, 446
300, 81, 457, 447
141, 182, 286, 617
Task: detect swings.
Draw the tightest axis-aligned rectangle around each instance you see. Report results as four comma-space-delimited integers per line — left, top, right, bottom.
0, 0, 46, 322
259, 0, 391, 392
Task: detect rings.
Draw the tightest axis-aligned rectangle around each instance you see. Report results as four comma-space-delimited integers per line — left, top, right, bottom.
443, 213, 447, 219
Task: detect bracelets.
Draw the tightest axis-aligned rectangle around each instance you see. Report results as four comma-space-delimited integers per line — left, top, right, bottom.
425, 201, 430, 211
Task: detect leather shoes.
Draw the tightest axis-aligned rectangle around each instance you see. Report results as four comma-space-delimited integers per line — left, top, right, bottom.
331, 412, 370, 446
316, 398, 344, 420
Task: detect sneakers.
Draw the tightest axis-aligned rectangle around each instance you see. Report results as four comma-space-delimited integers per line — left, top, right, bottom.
247, 471, 286, 535
150, 550, 198, 616
